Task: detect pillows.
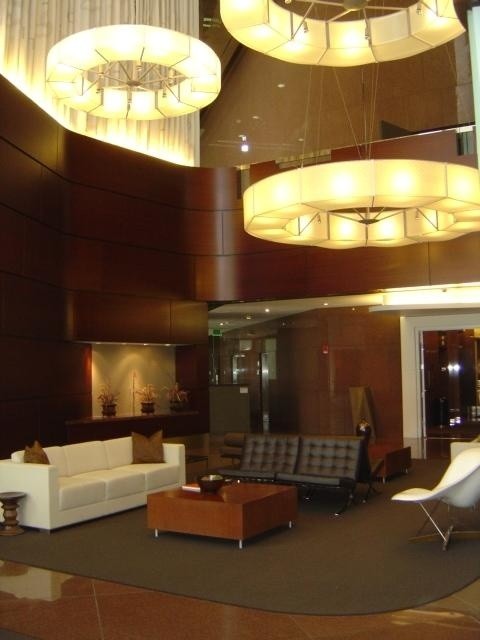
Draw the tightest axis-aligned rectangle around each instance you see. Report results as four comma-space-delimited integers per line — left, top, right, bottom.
24, 440, 50, 464
131, 428, 166, 464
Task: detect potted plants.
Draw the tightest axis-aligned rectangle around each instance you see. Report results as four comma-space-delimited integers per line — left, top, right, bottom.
163, 382, 193, 414
135, 383, 160, 417
97, 391, 121, 418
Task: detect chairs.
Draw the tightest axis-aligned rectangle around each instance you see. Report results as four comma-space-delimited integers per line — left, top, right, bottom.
390, 447, 480, 552
217, 433, 300, 504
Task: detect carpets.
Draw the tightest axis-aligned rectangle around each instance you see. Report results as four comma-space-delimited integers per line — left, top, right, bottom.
0, 459, 480, 616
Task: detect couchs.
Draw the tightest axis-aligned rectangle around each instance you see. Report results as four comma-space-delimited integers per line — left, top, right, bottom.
0, 436, 187, 533
275, 435, 367, 517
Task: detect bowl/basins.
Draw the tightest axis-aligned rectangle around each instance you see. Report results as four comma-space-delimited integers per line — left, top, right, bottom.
196, 474, 224, 493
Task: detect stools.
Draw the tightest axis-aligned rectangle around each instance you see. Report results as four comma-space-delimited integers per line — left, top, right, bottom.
0, 492, 27, 536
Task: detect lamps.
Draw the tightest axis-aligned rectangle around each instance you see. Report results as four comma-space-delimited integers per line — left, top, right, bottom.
220, 0, 467, 69
242, 0, 480, 250
45, 24, 222, 121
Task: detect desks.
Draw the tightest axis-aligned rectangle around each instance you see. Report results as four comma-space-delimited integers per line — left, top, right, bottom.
146, 482, 300, 550
367, 445, 410, 486
